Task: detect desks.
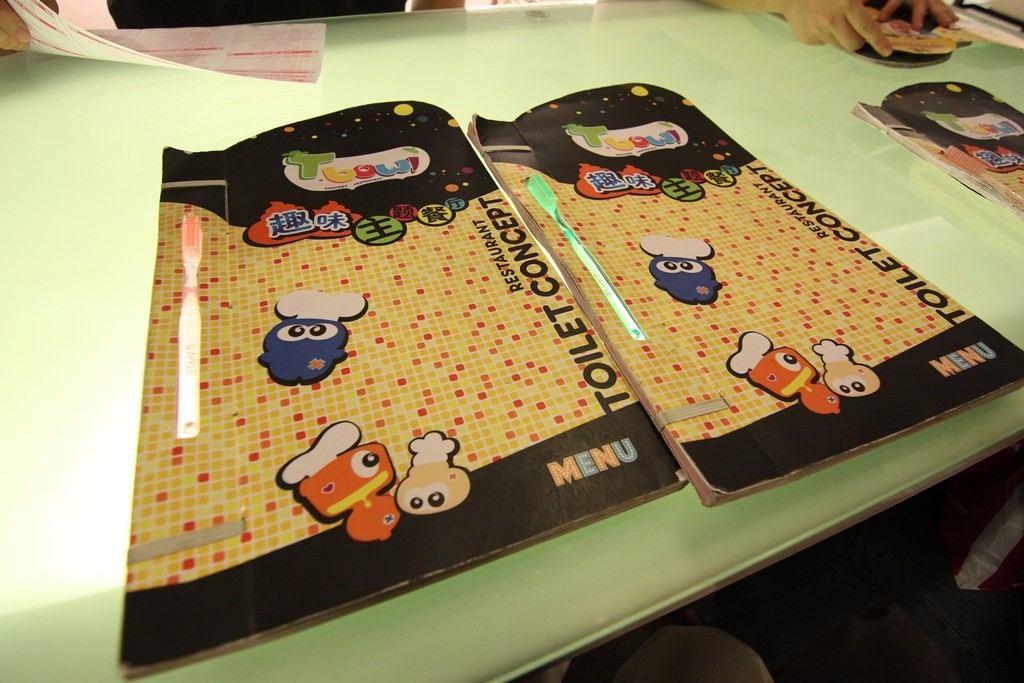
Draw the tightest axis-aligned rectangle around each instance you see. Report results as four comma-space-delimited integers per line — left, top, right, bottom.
0, 0, 1024, 683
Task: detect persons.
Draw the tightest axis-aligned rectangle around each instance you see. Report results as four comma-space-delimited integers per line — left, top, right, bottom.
0, 0, 466, 52
697, 0, 962, 57
610, 625, 775, 683
